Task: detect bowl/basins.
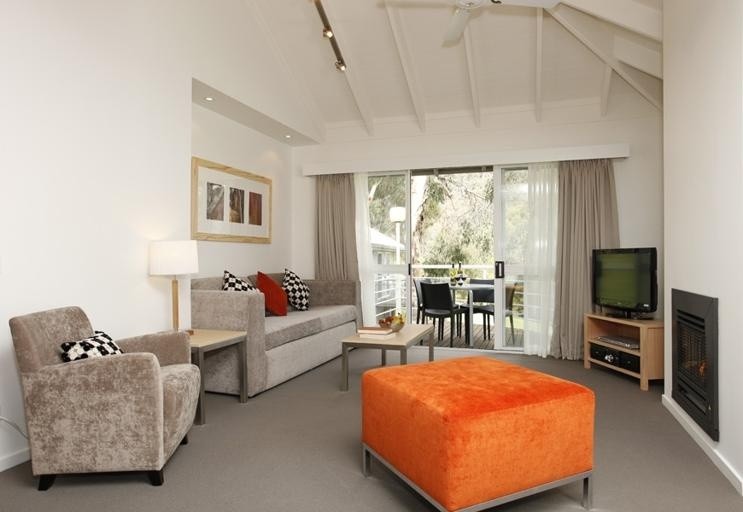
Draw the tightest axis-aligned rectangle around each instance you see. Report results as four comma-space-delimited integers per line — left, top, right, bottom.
379, 323, 404, 332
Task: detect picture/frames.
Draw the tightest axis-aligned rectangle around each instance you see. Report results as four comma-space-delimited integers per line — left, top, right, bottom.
190, 155, 272, 244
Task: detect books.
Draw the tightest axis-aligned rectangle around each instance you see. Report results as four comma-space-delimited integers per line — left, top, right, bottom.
357, 328, 393, 335
358, 333, 396, 340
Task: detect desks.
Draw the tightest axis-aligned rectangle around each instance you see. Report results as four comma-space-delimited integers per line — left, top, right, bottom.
449, 284, 493, 347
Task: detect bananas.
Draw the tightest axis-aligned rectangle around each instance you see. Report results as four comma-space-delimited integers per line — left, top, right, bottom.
393, 314, 402, 327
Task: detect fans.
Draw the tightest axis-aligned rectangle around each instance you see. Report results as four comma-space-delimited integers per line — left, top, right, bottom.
382, 1, 561, 43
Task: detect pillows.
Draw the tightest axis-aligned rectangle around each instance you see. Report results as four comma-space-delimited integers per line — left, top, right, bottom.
255, 271, 287, 316
281, 268, 310, 311
221, 270, 257, 291
60, 331, 124, 361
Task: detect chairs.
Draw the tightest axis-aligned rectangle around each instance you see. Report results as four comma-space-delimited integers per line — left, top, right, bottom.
412, 277, 443, 341
419, 281, 469, 348
479, 283, 517, 345
459, 279, 494, 340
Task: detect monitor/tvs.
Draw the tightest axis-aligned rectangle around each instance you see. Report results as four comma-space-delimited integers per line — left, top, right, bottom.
591, 247, 658, 320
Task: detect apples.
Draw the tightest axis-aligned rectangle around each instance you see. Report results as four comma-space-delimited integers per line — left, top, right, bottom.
378, 316, 392, 327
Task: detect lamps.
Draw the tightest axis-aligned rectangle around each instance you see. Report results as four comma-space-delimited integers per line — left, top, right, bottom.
148, 239, 198, 331
323, 29, 333, 38
334, 61, 346, 71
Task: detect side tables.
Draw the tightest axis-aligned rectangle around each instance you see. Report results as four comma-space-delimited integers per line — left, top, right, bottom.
184, 329, 248, 425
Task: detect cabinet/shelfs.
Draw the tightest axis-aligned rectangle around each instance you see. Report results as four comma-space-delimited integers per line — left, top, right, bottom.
583, 313, 665, 392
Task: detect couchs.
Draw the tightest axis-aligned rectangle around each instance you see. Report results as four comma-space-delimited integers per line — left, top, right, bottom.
191, 272, 363, 397
9, 306, 201, 491
360, 355, 594, 512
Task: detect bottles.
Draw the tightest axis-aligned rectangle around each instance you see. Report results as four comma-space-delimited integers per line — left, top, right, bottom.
450, 263, 456, 286
457, 263, 463, 275
